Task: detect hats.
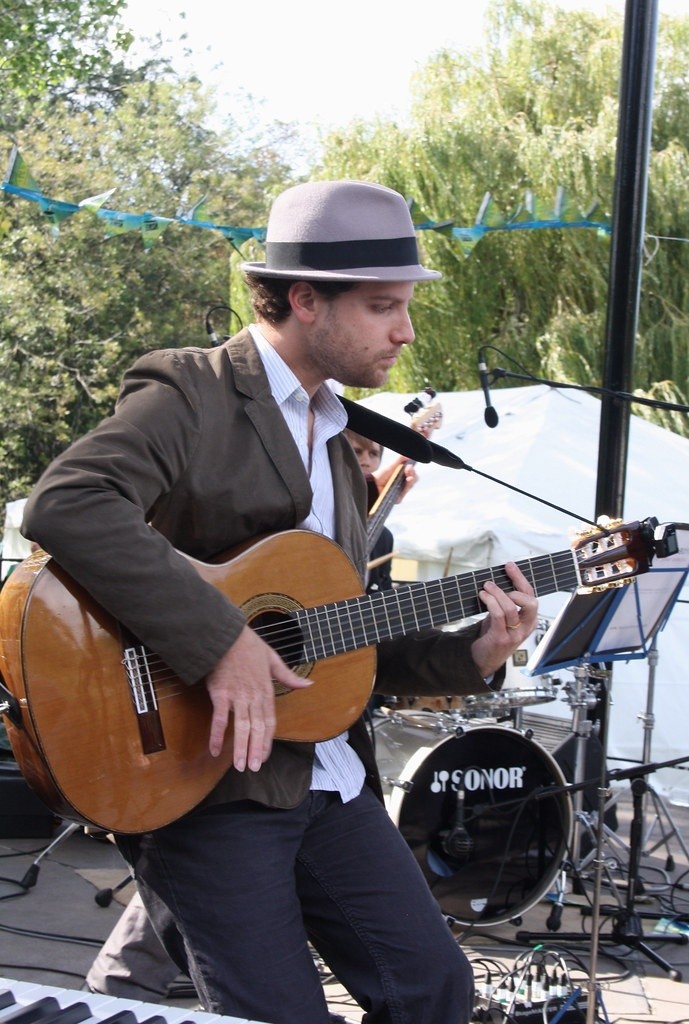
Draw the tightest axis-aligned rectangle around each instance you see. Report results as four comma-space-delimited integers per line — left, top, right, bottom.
239, 180, 443, 283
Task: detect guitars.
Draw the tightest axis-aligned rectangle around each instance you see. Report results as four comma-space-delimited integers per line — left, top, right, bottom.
366, 387, 444, 559
0, 515, 655, 834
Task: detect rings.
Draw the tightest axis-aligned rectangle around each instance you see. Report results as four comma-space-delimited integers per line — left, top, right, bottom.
506, 622, 522, 629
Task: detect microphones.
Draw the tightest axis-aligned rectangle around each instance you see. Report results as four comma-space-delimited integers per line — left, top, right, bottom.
479, 352, 499, 427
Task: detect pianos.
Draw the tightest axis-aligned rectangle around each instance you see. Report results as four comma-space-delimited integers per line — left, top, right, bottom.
0, 976, 275, 1024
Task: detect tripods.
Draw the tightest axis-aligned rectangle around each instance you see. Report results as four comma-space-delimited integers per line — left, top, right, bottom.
517, 566, 687, 982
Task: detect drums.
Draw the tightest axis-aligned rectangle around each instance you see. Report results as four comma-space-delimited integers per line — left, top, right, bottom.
458, 616, 556, 712
384, 694, 458, 729
388, 724, 574, 925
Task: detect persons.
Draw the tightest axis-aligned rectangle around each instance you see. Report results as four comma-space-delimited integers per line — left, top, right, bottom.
342, 427, 419, 597
17, 181, 539, 1024
